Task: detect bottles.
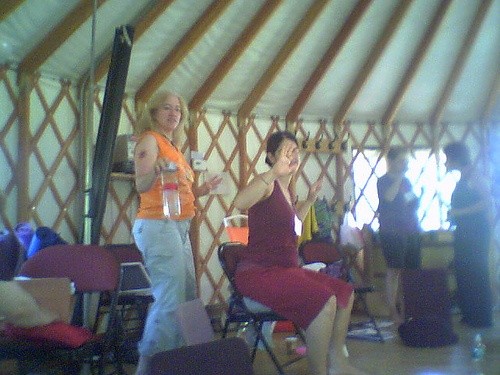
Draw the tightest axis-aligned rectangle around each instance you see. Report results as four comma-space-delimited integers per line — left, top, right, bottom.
469, 332, 488, 375
161, 162, 181, 216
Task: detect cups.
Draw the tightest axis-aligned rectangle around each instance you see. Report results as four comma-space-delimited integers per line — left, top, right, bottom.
286, 336, 298, 355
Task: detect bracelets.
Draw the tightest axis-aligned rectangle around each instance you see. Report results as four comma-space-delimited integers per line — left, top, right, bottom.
260, 176, 269, 185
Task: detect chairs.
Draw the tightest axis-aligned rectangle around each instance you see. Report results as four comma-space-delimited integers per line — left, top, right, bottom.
217, 241, 307, 375
298, 238, 386, 345
0, 243, 154, 375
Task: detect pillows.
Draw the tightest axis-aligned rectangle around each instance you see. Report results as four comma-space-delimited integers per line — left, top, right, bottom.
2, 320, 94, 350
0, 280, 60, 329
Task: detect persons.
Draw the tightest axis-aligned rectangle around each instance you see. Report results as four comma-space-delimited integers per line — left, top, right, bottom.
234, 131, 367, 375
133, 90, 223, 375
377, 146, 423, 321
443, 143, 493, 329
